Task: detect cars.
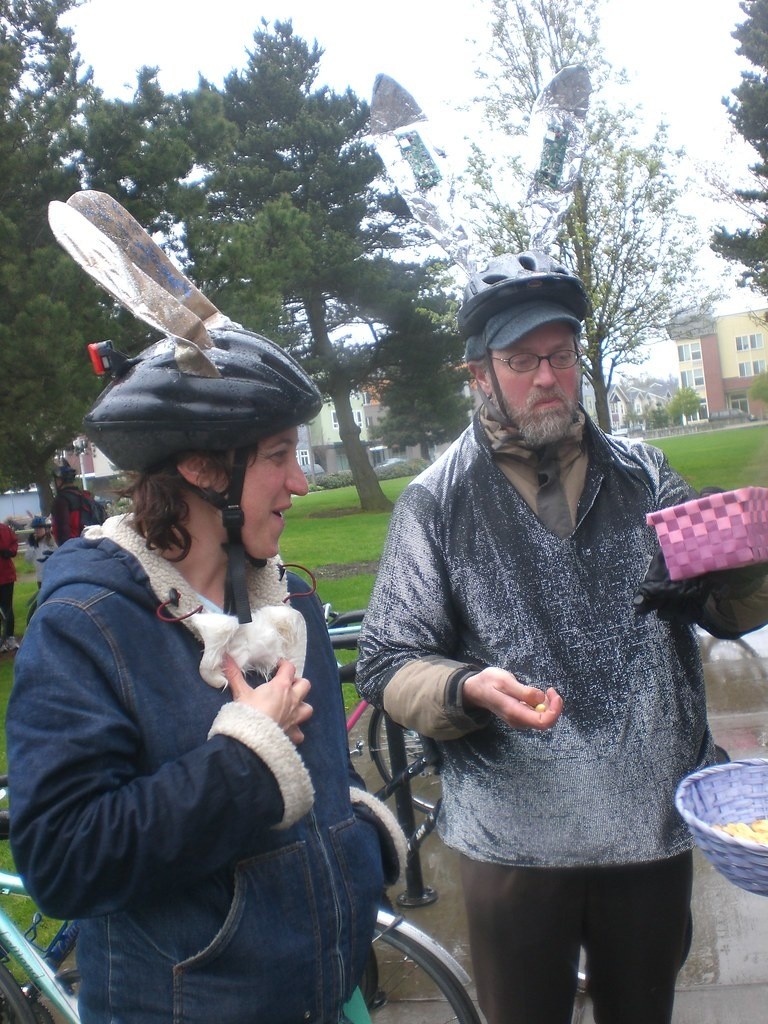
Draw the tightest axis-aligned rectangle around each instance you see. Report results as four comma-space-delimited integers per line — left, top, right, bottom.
614, 428, 629, 438
378, 457, 407, 467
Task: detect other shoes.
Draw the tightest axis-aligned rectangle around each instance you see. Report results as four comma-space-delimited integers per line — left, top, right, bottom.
0, 636, 20, 654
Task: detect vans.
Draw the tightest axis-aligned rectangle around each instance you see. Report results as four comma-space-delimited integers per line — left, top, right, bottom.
299, 465, 326, 476
709, 408, 757, 429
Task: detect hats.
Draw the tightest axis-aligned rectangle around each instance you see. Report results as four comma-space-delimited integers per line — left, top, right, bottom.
463, 300, 584, 365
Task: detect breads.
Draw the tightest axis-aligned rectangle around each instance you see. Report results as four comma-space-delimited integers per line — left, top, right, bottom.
711, 818, 768, 847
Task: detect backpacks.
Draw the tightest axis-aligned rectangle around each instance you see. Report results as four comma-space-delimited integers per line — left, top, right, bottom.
63, 486, 109, 535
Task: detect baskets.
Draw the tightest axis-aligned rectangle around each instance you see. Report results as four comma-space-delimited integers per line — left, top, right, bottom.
672, 757, 768, 898
644, 485, 768, 579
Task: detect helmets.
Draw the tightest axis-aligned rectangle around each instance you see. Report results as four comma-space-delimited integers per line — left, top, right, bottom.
46, 187, 325, 475
368, 65, 592, 340
50, 456, 77, 480
26, 509, 52, 528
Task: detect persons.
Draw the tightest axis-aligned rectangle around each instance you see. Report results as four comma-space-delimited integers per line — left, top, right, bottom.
24, 515, 59, 590
0, 524, 21, 654
51, 466, 107, 548
5, 322, 406, 1024
354, 252, 768, 1024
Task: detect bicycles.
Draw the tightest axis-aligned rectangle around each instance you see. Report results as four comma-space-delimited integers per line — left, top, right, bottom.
321, 603, 446, 851
0, 775, 479, 1023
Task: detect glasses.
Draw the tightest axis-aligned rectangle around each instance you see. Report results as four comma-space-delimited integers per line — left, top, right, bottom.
490, 350, 583, 372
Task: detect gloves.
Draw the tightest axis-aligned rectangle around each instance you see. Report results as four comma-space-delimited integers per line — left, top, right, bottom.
0, 549, 12, 559
632, 487, 727, 636
28, 533, 35, 546
36, 555, 48, 563
